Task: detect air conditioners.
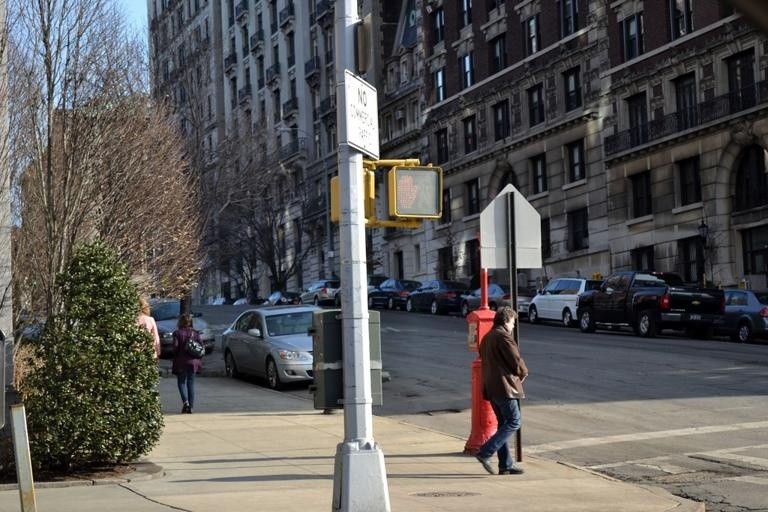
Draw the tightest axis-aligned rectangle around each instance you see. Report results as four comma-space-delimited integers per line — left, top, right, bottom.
395, 110, 404, 120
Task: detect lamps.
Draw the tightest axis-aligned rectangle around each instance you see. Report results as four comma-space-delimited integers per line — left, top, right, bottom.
698, 216, 712, 249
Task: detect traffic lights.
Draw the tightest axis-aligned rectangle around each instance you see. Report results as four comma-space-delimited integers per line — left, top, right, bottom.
389, 165, 443, 219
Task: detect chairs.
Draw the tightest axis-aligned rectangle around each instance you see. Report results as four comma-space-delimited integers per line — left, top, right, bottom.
266, 317, 311, 336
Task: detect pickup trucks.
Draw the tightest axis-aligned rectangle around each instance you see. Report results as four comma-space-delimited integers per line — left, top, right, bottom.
576, 271, 725, 337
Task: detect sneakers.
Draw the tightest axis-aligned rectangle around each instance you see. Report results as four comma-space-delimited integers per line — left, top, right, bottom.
181, 402, 190, 413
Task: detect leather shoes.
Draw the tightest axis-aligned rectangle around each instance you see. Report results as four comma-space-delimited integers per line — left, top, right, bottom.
499, 464, 524, 475
475, 452, 497, 475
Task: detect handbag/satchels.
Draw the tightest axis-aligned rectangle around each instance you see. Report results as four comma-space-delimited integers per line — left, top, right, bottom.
185, 330, 206, 359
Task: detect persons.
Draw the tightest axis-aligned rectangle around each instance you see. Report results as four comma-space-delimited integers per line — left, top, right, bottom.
474, 305, 527, 474
135, 299, 162, 363
170, 313, 202, 413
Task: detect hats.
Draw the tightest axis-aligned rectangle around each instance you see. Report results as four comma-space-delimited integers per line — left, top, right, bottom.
140, 299, 149, 309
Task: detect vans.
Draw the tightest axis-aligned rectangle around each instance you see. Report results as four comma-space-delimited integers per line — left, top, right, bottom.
528, 278, 630, 329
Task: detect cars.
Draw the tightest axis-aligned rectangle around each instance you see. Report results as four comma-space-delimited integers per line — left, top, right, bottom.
221, 305, 327, 389
406, 280, 470, 314
148, 299, 215, 359
262, 290, 301, 305
334, 275, 390, 308
460, 284, 535, 319
368, 278, 422, 309
298, 279, 340, 306
680, 290, 768, 342
